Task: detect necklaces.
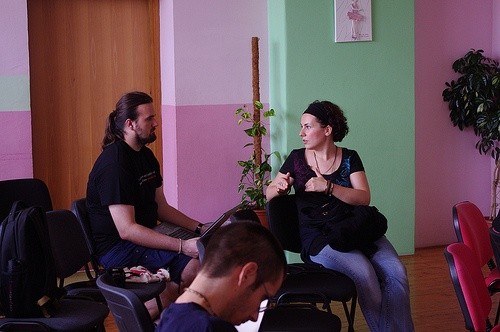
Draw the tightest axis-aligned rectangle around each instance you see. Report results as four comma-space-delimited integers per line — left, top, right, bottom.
313, 146, 337, 173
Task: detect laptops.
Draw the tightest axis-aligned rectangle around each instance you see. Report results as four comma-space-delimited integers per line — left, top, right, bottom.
152, 200, 248, 240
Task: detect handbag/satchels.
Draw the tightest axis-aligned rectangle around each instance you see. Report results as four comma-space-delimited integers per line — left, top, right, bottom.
298, 202, 387, 252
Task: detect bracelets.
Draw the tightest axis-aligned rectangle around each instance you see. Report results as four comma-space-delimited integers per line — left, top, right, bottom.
195, 223, 204, 235
178, 239, 182, 254
277, 187, 291, 195
325, 180, 333, 196
183, 288, 217, 317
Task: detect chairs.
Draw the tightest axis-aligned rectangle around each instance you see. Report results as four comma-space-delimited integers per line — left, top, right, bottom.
0, 178, 357, 332
444, 201, 500, 332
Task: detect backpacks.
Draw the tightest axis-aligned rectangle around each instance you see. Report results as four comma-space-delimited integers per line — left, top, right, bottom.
0, 200, 58, 318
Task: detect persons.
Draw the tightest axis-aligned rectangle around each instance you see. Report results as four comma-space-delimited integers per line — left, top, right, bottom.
156, 221, 287, 332
266, 99, 414, 332
85, 91, 213, 323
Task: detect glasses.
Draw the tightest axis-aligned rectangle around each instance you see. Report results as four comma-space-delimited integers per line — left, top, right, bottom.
256, 276, 277, 312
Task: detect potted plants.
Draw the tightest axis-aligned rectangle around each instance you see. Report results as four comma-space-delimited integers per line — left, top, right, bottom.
234, 102, 281, 229
442, 48, 500, 228
